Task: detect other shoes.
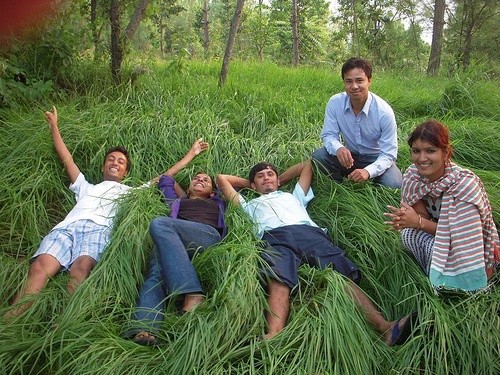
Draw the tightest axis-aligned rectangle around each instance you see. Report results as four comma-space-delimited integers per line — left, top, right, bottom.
179, 309, 187, 316
132, 331, 159, 345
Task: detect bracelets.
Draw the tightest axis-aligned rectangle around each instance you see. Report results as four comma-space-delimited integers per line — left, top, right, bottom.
416, 214, 423, 228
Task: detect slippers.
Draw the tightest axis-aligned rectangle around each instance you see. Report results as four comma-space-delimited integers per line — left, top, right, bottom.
390, 310, 420, 346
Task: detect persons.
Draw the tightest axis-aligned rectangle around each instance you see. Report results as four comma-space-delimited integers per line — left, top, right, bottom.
121, 171, 247, 344
311, 56, 403, 188
217, 161, 417, 347
4, 104, 208, 324
383, 120, 499, 296
